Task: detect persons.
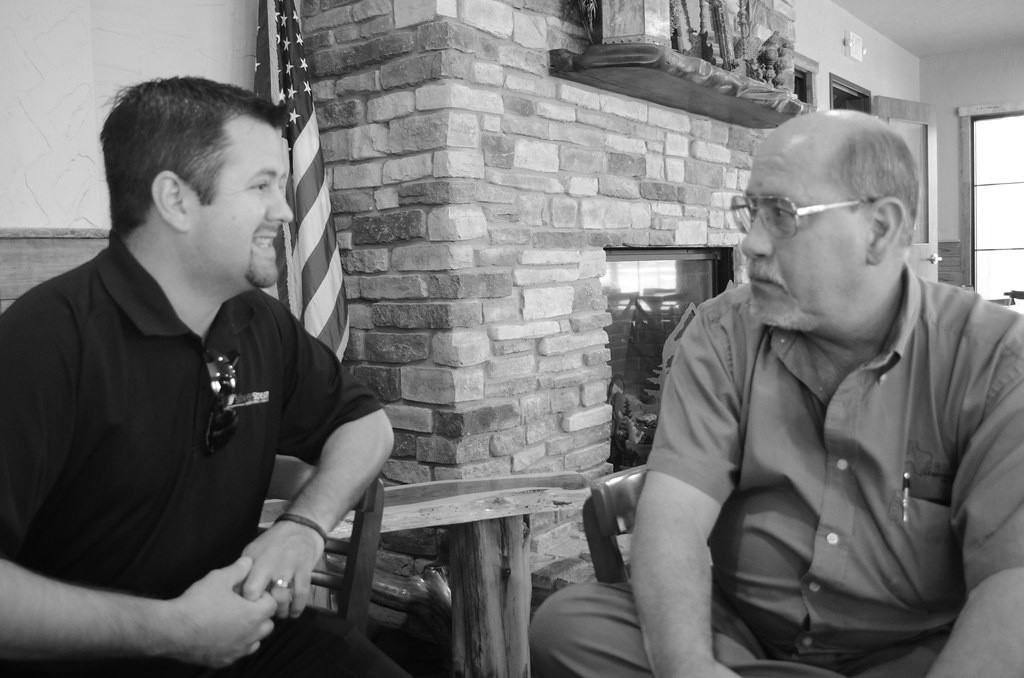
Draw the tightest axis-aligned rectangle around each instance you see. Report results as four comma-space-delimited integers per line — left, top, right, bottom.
529, 109, 1024, 678
2, 76, 405, 678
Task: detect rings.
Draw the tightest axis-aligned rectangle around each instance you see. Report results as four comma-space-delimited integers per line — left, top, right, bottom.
273, 580, 289, 587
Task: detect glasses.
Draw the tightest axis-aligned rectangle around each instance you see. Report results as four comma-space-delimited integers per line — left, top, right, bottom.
730, 195, 875, 239
204, 349, 240, 452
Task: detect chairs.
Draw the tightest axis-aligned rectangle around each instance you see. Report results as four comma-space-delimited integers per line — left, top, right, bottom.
580, 464, 645, 589
256, 454, 386, 643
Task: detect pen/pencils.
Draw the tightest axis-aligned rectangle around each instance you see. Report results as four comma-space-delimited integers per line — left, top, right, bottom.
902, 471, 913, 523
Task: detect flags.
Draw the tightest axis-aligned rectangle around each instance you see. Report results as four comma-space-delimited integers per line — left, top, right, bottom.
255, 0, 349, 365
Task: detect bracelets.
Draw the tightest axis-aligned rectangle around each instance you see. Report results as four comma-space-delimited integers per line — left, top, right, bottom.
275, 513, 326, 544
